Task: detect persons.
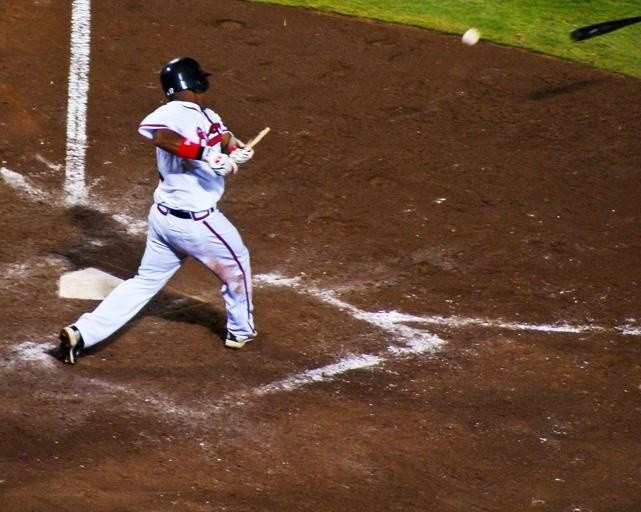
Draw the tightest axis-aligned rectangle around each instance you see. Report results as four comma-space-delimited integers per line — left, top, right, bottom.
58, 54, 259, 368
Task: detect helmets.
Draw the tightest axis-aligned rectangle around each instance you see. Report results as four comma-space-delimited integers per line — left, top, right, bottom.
159, 56, 213, 103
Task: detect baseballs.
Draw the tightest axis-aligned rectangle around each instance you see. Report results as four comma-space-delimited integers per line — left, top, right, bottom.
461, 30, 478, 46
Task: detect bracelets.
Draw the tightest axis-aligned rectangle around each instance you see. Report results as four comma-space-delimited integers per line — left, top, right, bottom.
176, 136, 202, 160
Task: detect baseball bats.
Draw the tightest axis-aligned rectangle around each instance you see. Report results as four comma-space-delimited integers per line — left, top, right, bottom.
570, 15, 641, 41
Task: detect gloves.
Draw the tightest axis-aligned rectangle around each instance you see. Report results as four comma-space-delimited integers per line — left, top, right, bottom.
228, 137, 255, 164
201, 145, 239, 177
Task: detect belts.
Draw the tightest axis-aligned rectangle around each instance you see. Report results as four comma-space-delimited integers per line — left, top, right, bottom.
156, 204, 215, 220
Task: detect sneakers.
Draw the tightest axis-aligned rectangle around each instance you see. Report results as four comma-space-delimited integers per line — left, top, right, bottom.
225, 331, 258, 349
60, 324, 85, 365
